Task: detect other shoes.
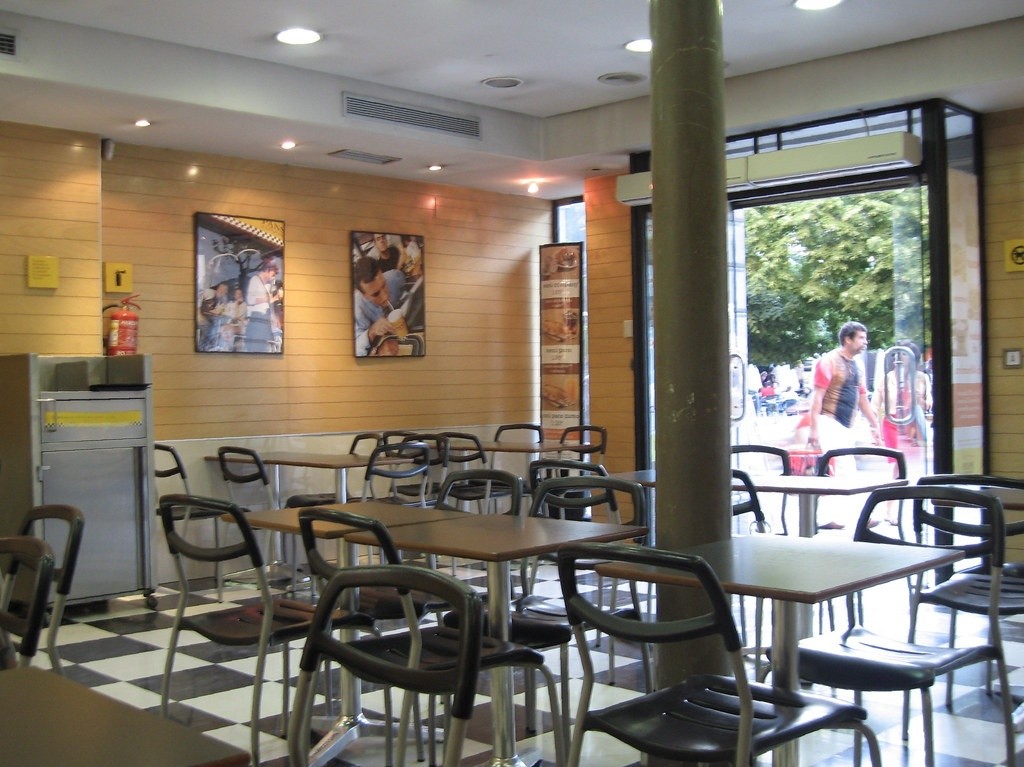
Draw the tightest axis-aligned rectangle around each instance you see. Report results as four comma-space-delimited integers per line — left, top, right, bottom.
867, 520, 880, 529
886, 519, 898, 526
818, 522, 845, 529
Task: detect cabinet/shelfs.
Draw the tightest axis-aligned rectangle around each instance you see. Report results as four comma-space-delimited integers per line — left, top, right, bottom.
2, 355, 160, 623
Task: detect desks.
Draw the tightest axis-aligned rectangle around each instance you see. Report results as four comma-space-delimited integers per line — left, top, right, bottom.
344, 513, 650, 767
414, 440, 593, 508
502, 504, 502, 508
220, 500, 485, 767
0, 667, 251, 767
594, 532, 965, 767
606, 469, 908, 538
205, 450, 414, 586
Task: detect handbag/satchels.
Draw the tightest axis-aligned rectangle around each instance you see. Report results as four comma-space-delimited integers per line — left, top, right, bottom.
788, 440, 835, 477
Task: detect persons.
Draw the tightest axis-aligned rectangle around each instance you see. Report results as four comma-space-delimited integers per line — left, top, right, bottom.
198, 262, 284, 352
353, 233, 421, 356
808, 321, 882, 530
870, 340, 933, 525
760, 359, 804, 415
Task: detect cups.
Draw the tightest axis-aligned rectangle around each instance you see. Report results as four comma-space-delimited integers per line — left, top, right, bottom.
385, 315, 410, 343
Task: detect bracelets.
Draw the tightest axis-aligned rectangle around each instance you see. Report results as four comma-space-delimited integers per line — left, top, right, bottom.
870, 422, 879, 432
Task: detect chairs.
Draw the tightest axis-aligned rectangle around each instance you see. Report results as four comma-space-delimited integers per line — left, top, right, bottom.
0, 423, 1024, 767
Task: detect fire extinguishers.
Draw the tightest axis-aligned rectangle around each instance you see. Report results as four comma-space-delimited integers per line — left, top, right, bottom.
102, 294, 143, 356
